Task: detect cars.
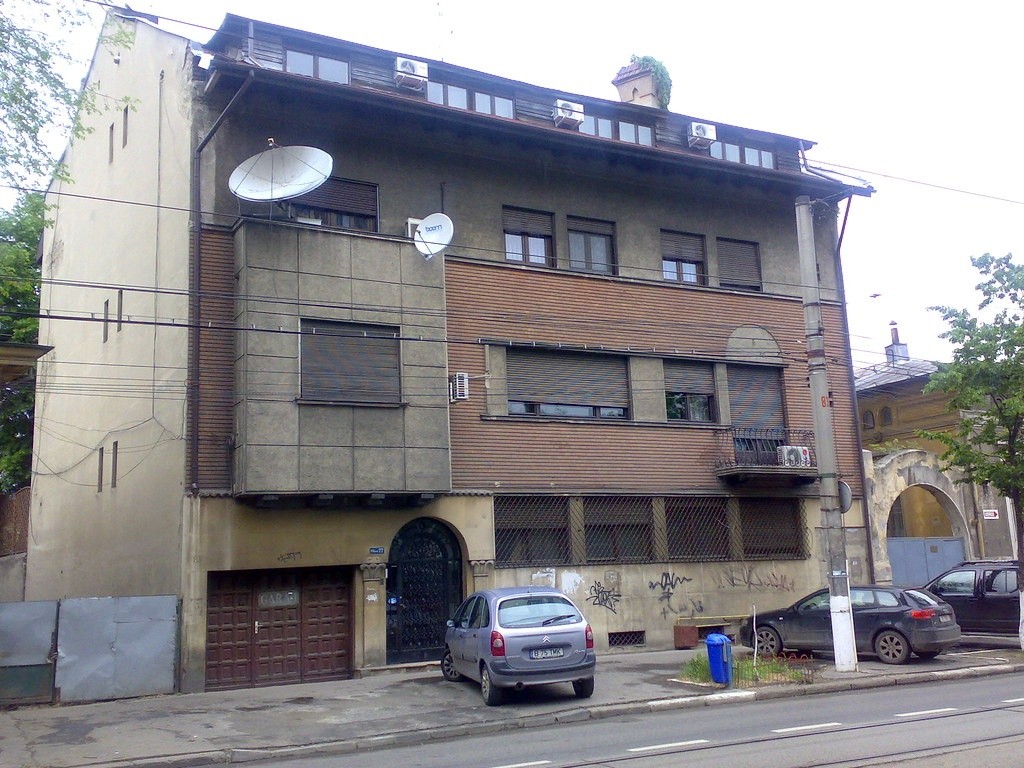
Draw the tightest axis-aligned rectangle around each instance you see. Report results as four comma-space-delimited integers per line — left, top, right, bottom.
441, 586, 596, 706
739, 584, 961, 665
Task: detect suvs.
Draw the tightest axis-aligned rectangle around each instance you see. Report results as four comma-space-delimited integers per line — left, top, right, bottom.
915, 557, 1020, 635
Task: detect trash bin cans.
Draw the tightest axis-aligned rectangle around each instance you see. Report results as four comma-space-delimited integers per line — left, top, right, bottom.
704, 633, 732, 684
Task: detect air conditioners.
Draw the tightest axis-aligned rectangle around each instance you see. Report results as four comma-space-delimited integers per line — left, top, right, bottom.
393, 57, 428, 88
688, 122, 717, 147
450, 373, 469, 400
553, 99, 584, 127
777, 446, 811, 467
